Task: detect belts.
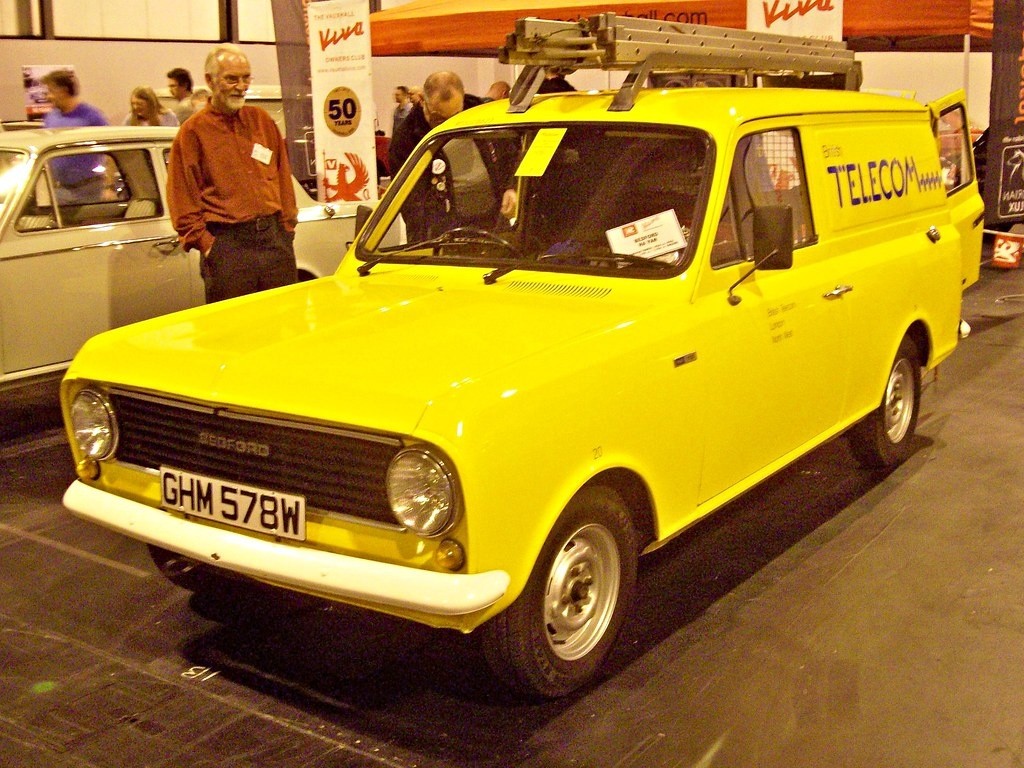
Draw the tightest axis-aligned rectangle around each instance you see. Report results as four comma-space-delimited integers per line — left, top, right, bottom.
61, 175, 102, 189
213, 212, 279, 231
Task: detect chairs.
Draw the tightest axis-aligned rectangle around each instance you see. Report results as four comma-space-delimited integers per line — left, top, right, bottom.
124, 197, 160, 220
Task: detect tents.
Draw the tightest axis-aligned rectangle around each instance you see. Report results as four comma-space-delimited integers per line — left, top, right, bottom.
371, 0, 997, 184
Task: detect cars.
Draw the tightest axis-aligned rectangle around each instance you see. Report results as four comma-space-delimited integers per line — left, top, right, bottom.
0, 129, 404, 398
62, 86, 985, 705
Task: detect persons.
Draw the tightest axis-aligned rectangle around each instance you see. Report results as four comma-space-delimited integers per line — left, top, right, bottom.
166, 67, 212, 125
388, 64, 579, 246
122, 88, 181, 127
165, 43, 297, 300
40, 71, 111, 208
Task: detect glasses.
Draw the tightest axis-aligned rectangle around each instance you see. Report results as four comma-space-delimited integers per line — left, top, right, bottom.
425, 100, 447, 126
